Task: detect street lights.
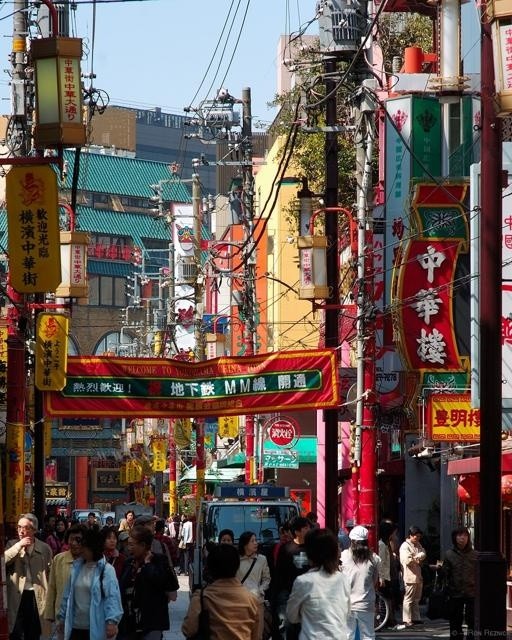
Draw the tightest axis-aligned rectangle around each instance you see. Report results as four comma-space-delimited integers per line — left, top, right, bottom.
298, 207, 365, 537
148, 416, 177, 518
127, 420, 151, 505
477, 3, 512, 640
6, 203, 91, 546
207, 316, 257, 487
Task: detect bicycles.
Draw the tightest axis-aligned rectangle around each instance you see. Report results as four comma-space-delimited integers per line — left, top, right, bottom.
374, 591, 390, 632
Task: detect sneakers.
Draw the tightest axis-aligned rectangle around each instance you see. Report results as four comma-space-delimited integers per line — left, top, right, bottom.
412, 620, 424, 627
402, 622, 414, 626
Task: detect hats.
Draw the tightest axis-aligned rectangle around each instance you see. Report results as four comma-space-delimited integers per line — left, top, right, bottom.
344, 519, 354, 527
133, 515, 157, 526
348, 525, 369, 541
106, 516, 113, 521
379, 518, 400, 537
408, 526, 423, 536
118, 531, 130, 542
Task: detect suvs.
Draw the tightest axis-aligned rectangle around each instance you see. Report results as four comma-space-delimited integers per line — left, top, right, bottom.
71, 509, 103, 526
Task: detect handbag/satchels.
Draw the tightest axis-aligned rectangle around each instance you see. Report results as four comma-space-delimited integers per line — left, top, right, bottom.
178, 524, 187, 549
426, 565, 464, 621
186, 588, 210, 640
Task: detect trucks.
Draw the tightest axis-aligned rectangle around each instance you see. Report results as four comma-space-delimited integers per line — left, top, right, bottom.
188, 500, 300, 604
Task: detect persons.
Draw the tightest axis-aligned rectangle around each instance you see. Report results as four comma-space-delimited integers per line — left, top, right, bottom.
118, 525, 179, 639
181, 543, 264, 640
273, 523, 291, 567
134, 515, 177, 604
83, 511, 102, 530
286, 528, 351, 640
165, 512, 195, 575
305, 512, 318, 529
276, 519, 293, 565
339, 525, 386, 640
378, 523, 406, 630
104, 516, 117, 529
384, 520, 404, 619
266, 515, 312, 640
441, 526, 482, 640
218, 529, 234, 545
235, 531, 271, 640
43, 524, 89, 626
50, 532, 124, 640
98, 527, 127, 578
118, 509, 137, 531
338, 519, 355, 551
3, 513, 52, 640
399, 525, 426, 627
151, 519, 174, 573
47, 517, 68, 557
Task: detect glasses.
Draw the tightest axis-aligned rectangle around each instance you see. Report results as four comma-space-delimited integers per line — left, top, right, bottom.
15, 524, 33, 530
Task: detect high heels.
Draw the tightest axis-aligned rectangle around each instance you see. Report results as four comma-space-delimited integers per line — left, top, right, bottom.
392, 623, 399, 630
387, 626, 393, 629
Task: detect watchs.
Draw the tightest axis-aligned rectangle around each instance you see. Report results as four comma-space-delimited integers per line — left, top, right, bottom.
105, 619, 117, 625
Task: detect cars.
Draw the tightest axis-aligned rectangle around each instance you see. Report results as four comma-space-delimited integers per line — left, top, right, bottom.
101, 511, 115, 525
